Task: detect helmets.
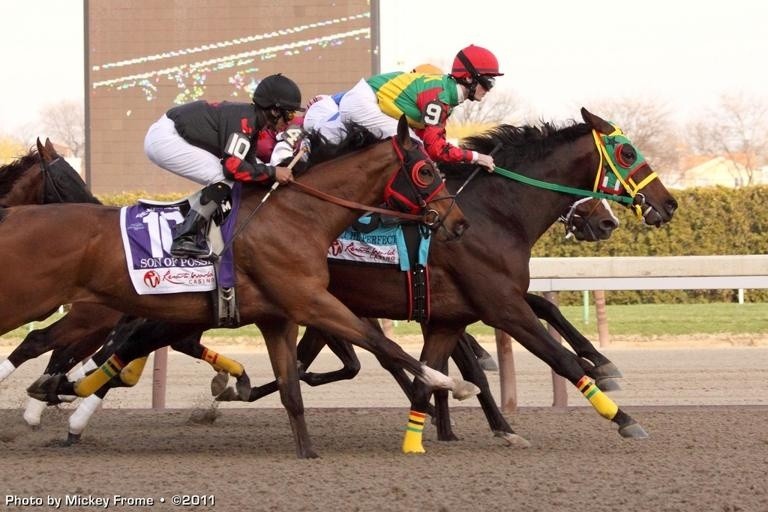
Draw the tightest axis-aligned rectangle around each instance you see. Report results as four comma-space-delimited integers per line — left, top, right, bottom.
409, 64, 443, 76
253, 74, 304, 111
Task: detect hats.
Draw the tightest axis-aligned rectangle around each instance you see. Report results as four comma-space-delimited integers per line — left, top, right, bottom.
451, 44, 505, 78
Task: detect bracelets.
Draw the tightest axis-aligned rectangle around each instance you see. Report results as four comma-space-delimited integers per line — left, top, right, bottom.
470, 150, 480, 165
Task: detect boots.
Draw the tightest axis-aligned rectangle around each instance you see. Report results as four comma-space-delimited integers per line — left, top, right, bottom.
171, 193, 220, 261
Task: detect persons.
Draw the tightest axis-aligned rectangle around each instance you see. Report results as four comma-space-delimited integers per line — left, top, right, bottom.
297, 61, 444, 152
338, 42, 506, 176
139, 72, 303, 267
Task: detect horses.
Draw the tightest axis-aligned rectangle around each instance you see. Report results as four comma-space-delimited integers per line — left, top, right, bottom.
0, 107, 678, 458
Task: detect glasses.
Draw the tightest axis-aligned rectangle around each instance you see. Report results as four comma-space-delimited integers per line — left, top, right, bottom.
259, 80, 294, 123
458, 49, 495, 91
